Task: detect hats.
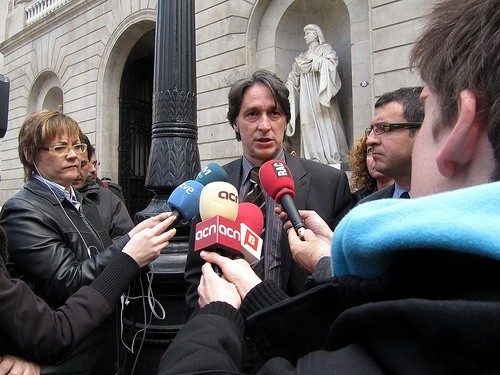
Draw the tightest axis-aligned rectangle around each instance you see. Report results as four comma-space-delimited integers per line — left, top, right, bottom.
97, 170, 112, 180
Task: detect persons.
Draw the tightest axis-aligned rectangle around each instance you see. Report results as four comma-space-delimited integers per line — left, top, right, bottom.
0, 0, 499, 375
285, 24, 349, 164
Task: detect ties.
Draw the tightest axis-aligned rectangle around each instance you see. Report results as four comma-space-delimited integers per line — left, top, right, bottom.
241, 168, 267, 281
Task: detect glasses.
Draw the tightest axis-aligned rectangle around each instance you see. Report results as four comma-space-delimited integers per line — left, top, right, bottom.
89, 161, 101, 171
38, 143, 87, 157
364, 122, 422, 136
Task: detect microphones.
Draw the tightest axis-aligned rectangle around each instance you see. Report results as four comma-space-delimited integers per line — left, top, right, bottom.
259, 160, 307, 241
159, 162, 264, 278
